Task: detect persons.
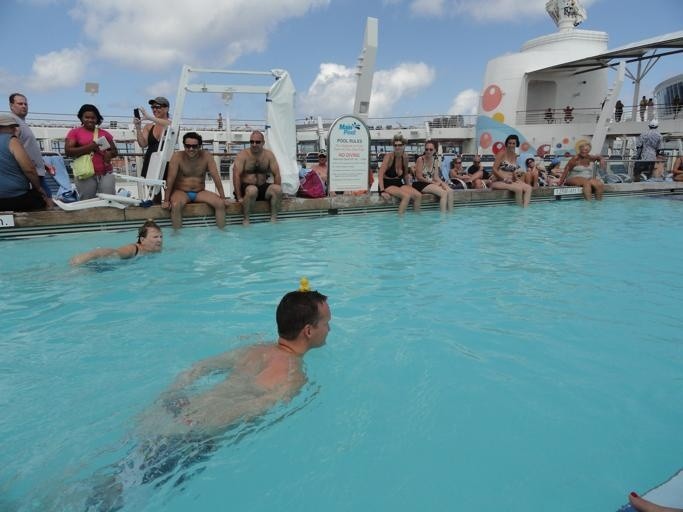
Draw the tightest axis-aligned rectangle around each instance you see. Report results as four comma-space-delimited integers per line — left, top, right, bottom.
628, 491, 683, 512
76, 291, 331, 512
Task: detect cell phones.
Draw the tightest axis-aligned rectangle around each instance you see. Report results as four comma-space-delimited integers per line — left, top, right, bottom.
134, 108, 140, 120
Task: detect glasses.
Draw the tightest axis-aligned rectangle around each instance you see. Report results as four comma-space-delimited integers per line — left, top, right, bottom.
250, 140, 264, 145
184, 143, 200, 149
425, 148, 435, 152
394, 144, 404, 147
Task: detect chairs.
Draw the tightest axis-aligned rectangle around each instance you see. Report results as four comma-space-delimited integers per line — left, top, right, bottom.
598, 168, 631, 185
40, 150, 79, 210
515, 153, 558, 187
438, 153, 486, 190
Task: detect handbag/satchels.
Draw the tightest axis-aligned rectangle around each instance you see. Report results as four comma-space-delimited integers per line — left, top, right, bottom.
69, 154, 95, 181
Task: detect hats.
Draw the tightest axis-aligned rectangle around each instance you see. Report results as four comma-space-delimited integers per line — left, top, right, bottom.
148, 96, 169, 108
0, 113, 19, 128
648, 119, 658, 128
318, 149, 328, 157
